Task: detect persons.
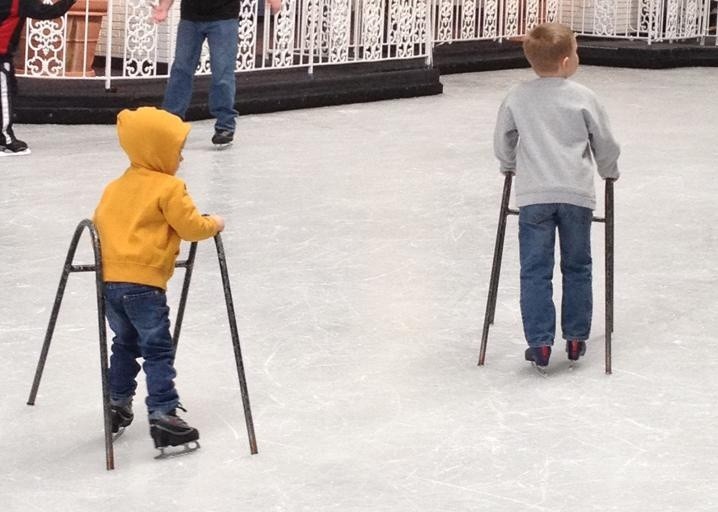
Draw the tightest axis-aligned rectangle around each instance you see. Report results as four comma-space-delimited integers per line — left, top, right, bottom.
92, 104, 226, 449
148, 0, 282, 145
492, 25, 621, 366
0, 0, 78, 154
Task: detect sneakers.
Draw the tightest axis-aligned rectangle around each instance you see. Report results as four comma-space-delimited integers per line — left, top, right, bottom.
524, 347, 551, 365
212, 128, 234, 144
565, 340, 586, 359
148, 408, 198, 441
1, 139, 28, 153
108, 396, 134, 426
